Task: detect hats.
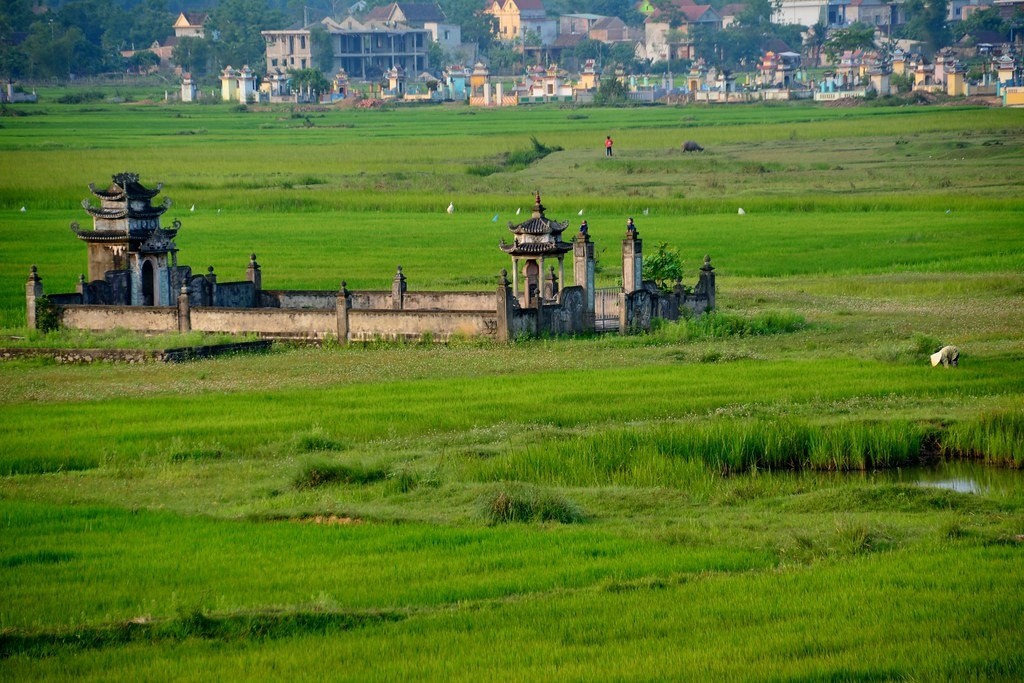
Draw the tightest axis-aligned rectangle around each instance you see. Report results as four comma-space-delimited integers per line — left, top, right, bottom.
930, 351, 942, 367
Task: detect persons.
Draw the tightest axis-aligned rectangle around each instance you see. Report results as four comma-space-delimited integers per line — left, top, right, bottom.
930, 346, 959, 368
605, 135, 614, 157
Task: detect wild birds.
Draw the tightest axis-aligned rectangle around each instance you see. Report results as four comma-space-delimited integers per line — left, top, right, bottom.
189, 204, 223, 215
446, 199, 745, 222
21, 206, 26, 212
